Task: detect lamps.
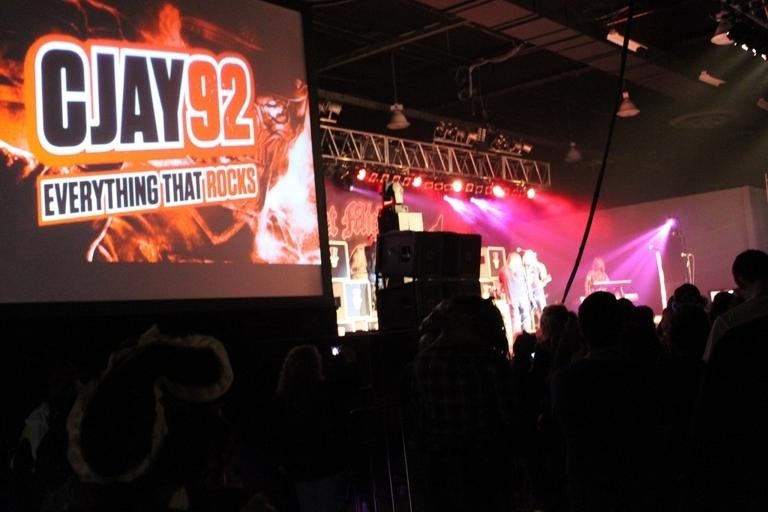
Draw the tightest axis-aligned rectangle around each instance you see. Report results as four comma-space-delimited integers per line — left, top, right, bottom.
316, 49, 536, 201
711, 13, 736, 45
617, 88, 641, 117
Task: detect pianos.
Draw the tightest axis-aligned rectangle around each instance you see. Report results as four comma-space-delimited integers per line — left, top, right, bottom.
592, 279, 632, 289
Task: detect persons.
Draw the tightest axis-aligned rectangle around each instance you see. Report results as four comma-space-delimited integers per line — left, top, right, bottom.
499, 249, 768, 363
365, 234, 383, 290
417, 297, 509, 358
